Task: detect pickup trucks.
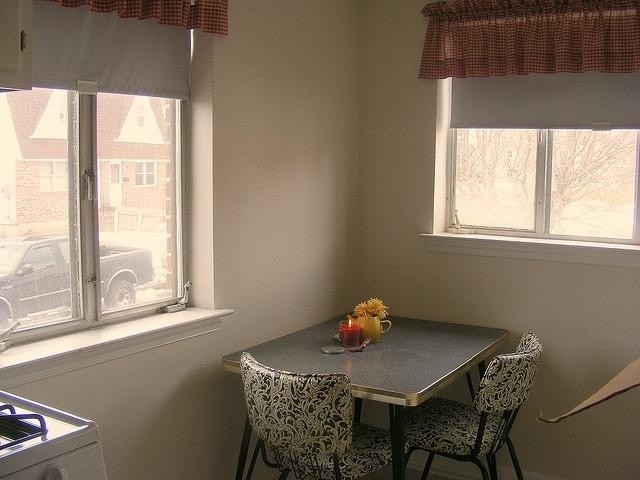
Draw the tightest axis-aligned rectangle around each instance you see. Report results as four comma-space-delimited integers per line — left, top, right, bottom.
1, 235, 154, 331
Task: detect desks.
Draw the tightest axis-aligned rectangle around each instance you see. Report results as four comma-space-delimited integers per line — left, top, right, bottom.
220, 311, 510, 477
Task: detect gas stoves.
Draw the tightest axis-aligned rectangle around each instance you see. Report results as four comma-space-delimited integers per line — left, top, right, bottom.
0, 388, 108, 480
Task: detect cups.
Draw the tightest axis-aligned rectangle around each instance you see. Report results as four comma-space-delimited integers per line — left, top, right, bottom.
339, 320, 362, 346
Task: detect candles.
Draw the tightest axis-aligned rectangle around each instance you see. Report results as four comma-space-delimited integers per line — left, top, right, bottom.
339, 325, 361, 346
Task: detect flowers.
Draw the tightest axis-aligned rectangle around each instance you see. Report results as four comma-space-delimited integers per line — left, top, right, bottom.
351, 296, 391, 319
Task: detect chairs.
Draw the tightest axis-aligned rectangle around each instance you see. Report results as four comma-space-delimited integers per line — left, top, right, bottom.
404, 330, 541, 479
240, 350, 397, 479
